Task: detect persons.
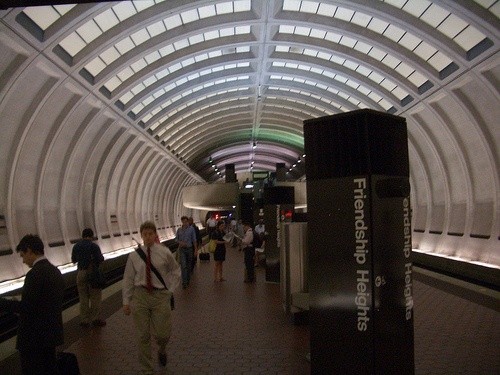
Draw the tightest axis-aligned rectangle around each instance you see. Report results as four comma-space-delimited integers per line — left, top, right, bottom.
72, 228, 107, 326
255, 219, 265, 235
122, 222, 181, 375
207, 214, 217, 239
210, 221, 229, 281
231, 219, 244, 251
9, 234, 66, 375
189, 217, 202, 272
175, 215, 198, 289
254, 232, 266, 266
236, 220, 256, 284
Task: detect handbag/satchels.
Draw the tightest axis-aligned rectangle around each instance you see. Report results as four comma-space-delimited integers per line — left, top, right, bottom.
87, 262, 104, 288
170, 295, 175, 311
54, 351, 80, 375
175, 248, 181, 264
208, 230, 219, 252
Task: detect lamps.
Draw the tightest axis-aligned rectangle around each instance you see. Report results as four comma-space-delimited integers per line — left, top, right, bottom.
285, 154, 306, 174
209, 156, 222, 177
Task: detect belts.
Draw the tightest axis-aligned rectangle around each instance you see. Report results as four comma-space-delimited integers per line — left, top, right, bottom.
141, 286, 160, 291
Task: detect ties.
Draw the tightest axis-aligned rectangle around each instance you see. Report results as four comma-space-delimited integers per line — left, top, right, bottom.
146, 248, 153, 292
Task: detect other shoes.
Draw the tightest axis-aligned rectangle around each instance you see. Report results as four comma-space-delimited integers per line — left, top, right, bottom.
80, 324, 90, 327
182, 283, 187, 289
157, 352, 167, 368
92, 320, 107, 327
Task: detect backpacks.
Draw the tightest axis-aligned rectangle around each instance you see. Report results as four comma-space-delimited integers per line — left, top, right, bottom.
250, 227, 263, 248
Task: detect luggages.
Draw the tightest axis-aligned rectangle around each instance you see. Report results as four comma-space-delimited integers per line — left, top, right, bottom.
199, 244, 210, 261
